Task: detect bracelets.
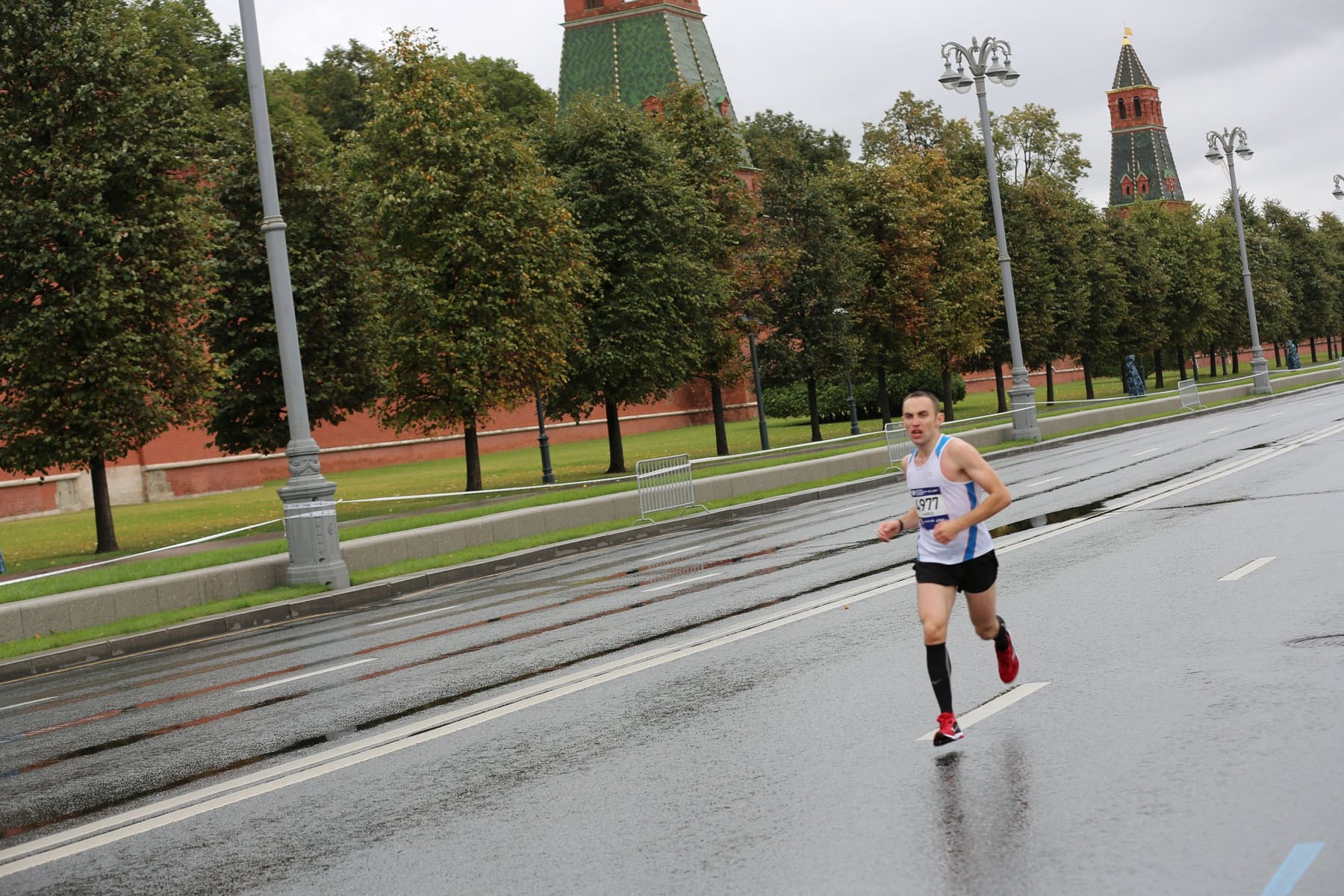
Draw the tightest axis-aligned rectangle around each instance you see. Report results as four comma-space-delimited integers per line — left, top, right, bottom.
897, 520, 905, 533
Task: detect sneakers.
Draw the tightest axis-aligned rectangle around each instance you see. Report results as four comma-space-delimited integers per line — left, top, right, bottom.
996, 634, 1020, 684
934, 713, 964, 746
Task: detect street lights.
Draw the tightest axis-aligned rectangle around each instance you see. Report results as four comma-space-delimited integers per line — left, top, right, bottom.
1205, 126, 1274, 394
934, 35, 1045, 442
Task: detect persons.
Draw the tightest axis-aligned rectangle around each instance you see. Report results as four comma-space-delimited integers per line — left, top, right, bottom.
877, 391, 1020, 746
